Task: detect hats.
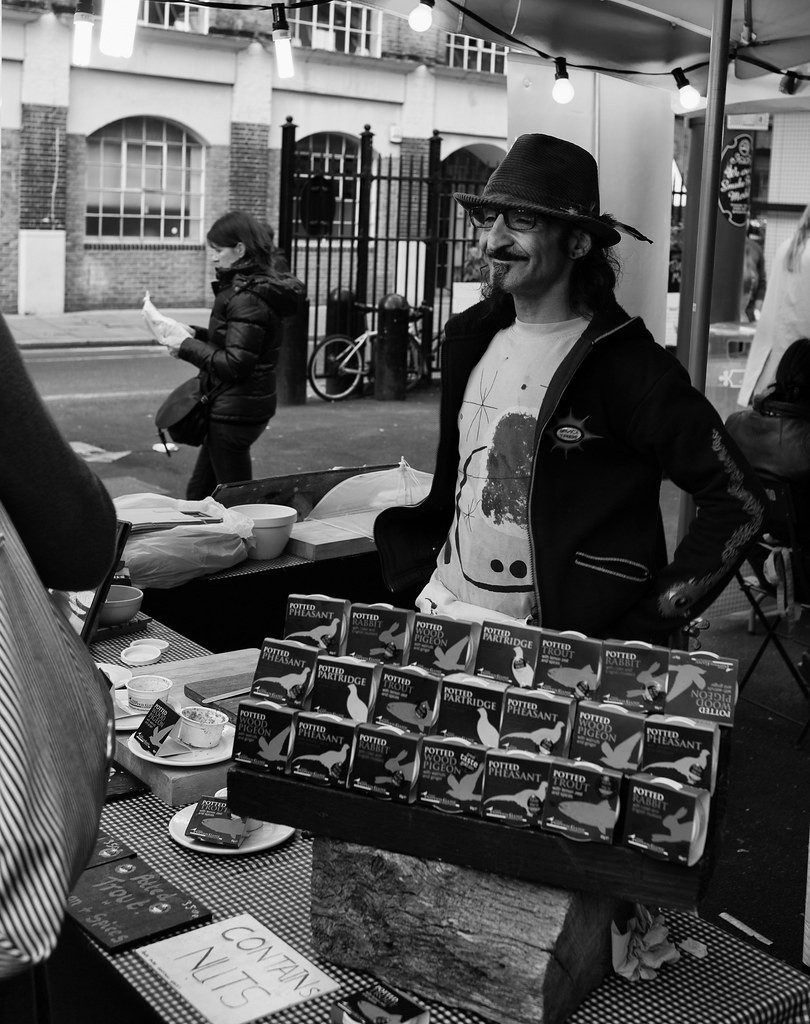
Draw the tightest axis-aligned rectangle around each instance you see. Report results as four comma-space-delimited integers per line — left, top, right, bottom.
454, 133, 655, 249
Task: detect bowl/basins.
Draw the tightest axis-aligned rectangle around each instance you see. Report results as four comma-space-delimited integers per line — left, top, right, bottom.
228, 504, 297, 560
75, 585, 144, 626
125, 675, 173, 712
178, 706, 229, 748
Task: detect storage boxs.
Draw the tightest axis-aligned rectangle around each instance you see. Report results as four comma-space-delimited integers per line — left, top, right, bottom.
234, 592, 741, 865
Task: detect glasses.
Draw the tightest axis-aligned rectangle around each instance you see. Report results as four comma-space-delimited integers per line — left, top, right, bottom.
466, 204, 574, 230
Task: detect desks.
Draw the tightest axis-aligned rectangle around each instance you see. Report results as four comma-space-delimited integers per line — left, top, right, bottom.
68, 600, 809, 1024
139, 459, 441, 631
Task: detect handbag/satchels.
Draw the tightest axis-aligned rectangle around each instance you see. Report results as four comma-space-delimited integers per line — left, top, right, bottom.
111, 492, 257, 589
0, 506, 114, 979
154, 377, 209, 448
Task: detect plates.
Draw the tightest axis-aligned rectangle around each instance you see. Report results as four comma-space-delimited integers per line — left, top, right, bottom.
169, 787, 295, 854
113, 689, 181, 730
127, 724, 235, 766
95, 662, 133, 689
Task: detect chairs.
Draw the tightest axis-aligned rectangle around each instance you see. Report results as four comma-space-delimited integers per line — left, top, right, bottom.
731, 468, 810, 703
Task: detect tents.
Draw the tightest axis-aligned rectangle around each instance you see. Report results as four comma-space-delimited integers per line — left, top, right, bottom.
349, 0, 810, 547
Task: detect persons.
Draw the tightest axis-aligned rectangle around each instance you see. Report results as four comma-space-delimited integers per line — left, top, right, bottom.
736, 202, 810, 408
168, 211, 308, 501
724, 338, 810, 684
373, 133, 771, 651
743, 227, 767, 322
0, 311, 118, 1024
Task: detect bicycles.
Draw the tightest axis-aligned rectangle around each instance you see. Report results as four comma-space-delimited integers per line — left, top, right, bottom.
308, 302, 433, 402
412, 329, 446, 387
